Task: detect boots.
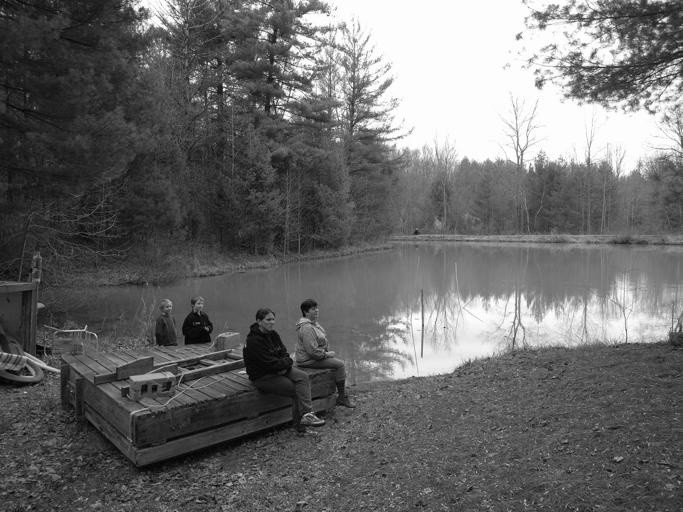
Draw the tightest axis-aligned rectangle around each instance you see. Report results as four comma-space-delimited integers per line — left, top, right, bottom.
333, 379, 356, 409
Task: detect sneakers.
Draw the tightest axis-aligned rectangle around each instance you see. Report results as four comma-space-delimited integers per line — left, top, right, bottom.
298, 428, 319, 437
298, 411, 326, 426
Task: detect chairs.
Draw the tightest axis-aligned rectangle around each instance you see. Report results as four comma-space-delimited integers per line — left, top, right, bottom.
42, 323, 100, 359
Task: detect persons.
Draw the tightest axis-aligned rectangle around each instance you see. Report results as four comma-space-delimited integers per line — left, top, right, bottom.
293, 297, 357, 409
154, 297, 179, 347
181, 295, 212, 344
240, 308, 325, 439
